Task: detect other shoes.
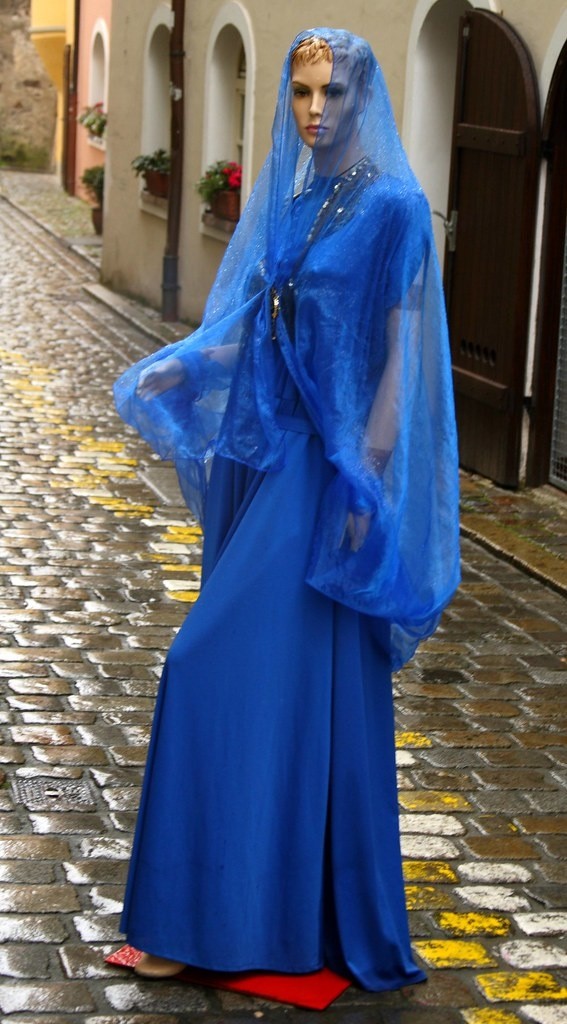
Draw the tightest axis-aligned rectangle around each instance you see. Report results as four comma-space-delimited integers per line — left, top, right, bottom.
136, 956, 185, 977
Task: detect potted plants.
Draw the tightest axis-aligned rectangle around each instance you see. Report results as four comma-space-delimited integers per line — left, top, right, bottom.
81, 165, 104, 236
130, 149, 172, 197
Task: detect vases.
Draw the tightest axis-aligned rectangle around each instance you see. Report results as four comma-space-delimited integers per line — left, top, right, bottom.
89, 129, 103, 137
206, 187, 240, 220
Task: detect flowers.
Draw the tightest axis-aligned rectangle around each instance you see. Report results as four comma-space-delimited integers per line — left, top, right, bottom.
199, 160, 242, 199
75, 103, 108, 131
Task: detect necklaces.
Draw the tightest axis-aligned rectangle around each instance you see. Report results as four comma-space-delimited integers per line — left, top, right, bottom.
259, 150, 379, 343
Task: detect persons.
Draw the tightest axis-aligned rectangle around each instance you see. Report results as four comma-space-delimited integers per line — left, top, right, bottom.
113, 24, 462, 991
